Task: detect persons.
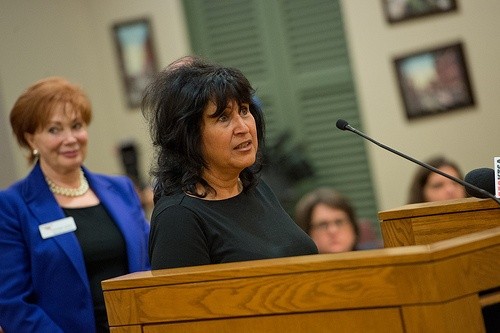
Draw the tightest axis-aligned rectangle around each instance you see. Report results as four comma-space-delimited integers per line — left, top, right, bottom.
138, 55, 319, 271
0, 76, 152, 328
293, 185, 362, 254
406, 155, 468, 204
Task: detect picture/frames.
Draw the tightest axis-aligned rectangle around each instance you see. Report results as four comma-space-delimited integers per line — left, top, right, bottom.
109, 17, 160, 108
393, 42, 475, 120
380, 0, 458, 24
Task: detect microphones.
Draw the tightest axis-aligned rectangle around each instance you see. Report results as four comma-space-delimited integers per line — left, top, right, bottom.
335, 118, 500, 205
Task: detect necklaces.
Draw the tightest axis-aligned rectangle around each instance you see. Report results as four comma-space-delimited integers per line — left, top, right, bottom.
46, 171, 90, 197
